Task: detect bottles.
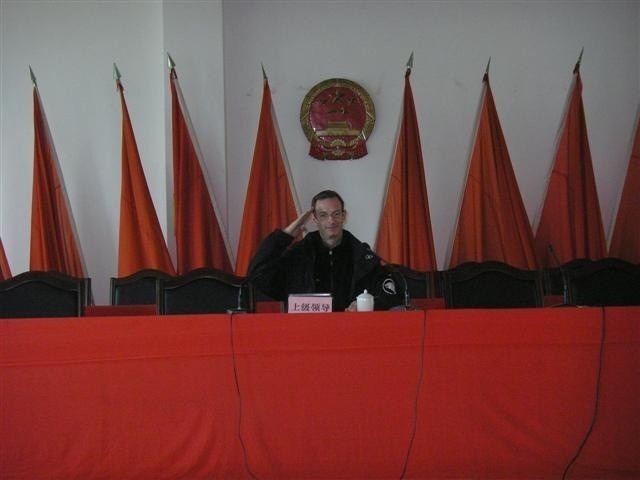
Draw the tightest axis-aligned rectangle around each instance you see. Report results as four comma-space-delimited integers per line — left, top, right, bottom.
357, 290, 375, 313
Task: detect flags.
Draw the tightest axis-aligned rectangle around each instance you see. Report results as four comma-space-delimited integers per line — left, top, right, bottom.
373, 48, 439, 272
234, 64, 304, 275
446, 57, 543, 275
110, 61, 178, 278
529, 48, 609, 268
167, 53, 235, 275
608, 104, 638, 266
27, 65, 90, 282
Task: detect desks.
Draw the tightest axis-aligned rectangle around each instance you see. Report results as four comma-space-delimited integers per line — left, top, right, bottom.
0, 304, 640, 480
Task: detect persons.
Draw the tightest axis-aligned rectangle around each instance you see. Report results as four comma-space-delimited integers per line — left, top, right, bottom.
247, 191, 416, 313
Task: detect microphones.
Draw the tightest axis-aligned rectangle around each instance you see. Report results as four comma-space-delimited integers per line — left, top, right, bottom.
389, 269, 416, 310
547, 243, 579, 307
228, 268, 263, 313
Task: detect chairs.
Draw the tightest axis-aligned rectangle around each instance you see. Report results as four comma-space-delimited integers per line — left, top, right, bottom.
0, 258, 640, 319
